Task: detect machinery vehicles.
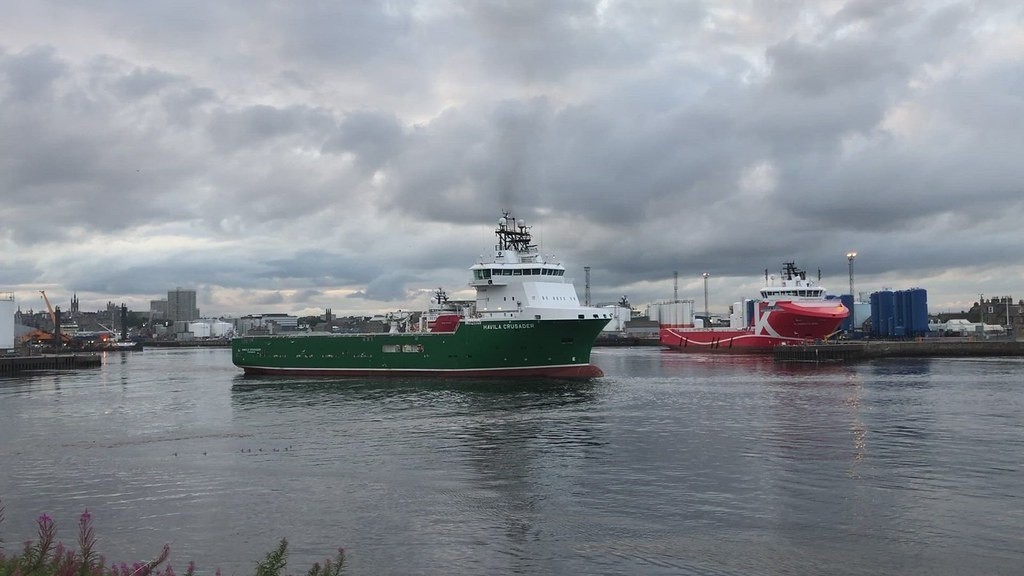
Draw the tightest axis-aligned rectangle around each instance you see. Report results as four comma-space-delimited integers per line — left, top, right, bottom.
22, 290, 71, 346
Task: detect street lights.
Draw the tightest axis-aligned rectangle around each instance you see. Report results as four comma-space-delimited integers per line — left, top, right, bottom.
846, 252, 856, 296
701, 272, 709, 316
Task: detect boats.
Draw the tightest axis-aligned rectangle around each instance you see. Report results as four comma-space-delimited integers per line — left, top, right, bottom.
231, 208, 614, 380
660, 258, 851, 345
